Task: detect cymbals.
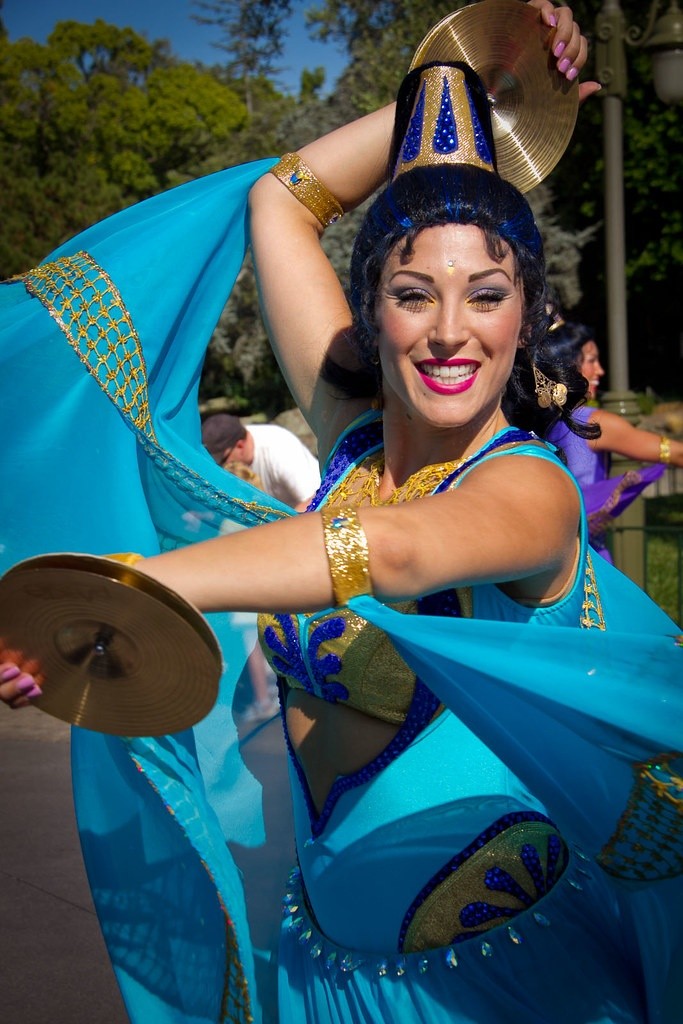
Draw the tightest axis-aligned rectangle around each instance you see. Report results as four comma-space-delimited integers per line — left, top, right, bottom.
408, 0, 580, 194
0, 553, 225, 738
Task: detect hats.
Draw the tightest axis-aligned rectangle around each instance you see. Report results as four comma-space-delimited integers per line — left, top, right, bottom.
201, 414, 246, 464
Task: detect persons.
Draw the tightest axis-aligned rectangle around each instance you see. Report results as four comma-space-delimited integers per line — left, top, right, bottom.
0, 0, 683, 1024
543, 320, 682, 563
200, 413, 322, 726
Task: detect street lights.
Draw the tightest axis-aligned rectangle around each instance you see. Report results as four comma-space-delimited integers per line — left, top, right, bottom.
585, 1, 683, 587
